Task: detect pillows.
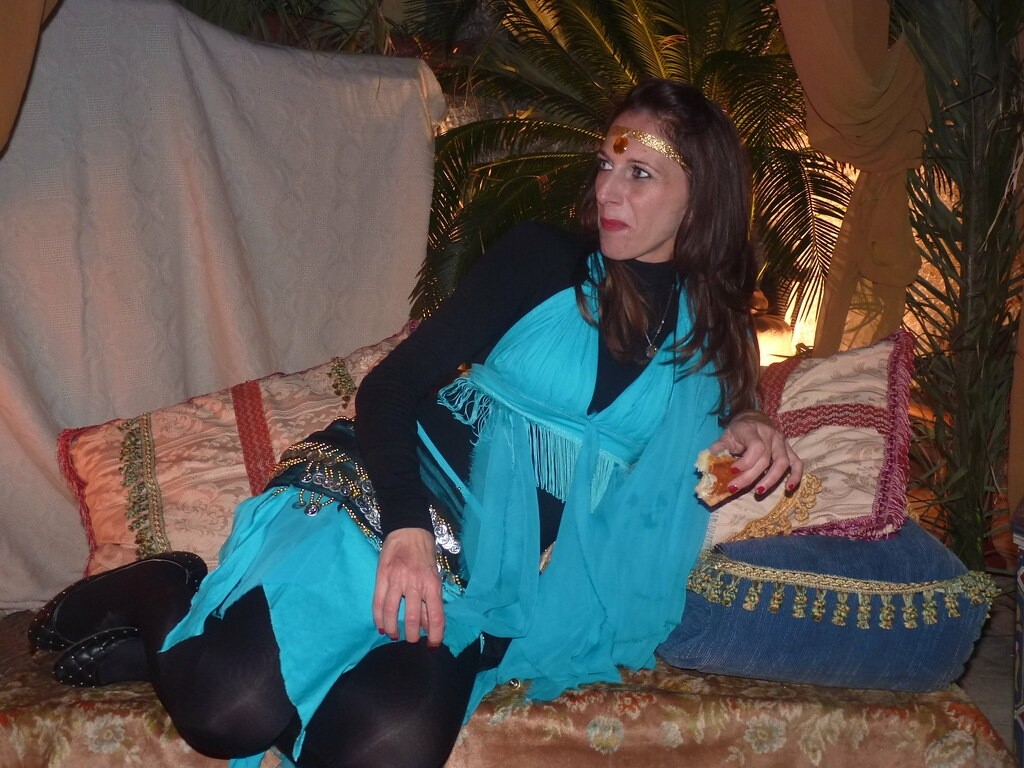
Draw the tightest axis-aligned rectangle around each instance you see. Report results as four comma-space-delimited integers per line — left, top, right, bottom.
714, 330, 916, 543
58, 320, 423, 571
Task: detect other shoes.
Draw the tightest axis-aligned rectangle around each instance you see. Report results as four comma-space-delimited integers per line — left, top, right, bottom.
29, 553, 208, 649
50, 627, 151, 686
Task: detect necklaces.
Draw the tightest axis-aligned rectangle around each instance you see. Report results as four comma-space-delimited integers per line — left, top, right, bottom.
644, 282, 674, 358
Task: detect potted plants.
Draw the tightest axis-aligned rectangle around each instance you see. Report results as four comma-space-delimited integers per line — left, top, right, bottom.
886, 0, 1024, 681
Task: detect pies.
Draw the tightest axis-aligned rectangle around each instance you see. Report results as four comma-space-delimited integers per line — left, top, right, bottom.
693, 450, 741, 506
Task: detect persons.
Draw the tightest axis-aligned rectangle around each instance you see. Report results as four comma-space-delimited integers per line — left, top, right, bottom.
28, 81, 800, 768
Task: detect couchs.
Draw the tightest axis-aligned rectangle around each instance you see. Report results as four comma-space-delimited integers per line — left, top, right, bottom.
1, 604, 1024, 768
